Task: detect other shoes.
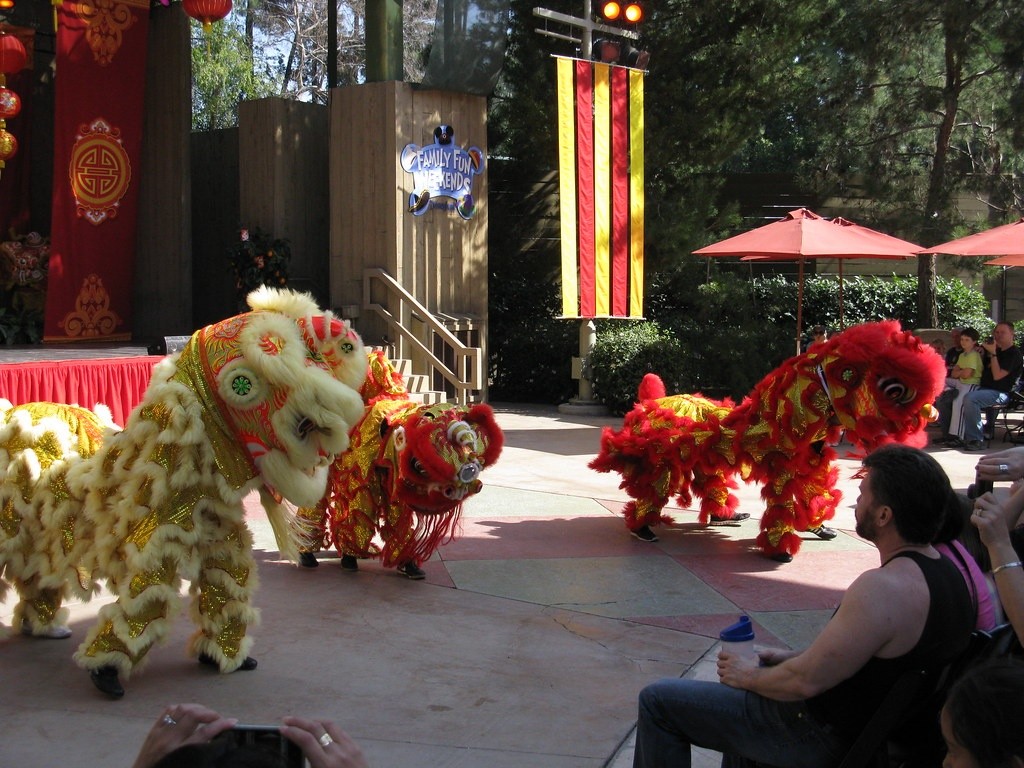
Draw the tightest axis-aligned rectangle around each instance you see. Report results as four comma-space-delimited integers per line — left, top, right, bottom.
91, 665, 125, 697
300, 552, 320, 568
941, 438, 967, 448
21, 618, 73, 639
932, 437, 958, 445
964, 439, 986, 451
198, 651, 258, 671
928, 421, 940, 427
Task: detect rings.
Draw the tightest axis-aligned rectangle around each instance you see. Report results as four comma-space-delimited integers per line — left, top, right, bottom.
977, 509, 982, 517
319, 733, 333, 747
1000, 465, 1008, 474
163, 714, 176, 726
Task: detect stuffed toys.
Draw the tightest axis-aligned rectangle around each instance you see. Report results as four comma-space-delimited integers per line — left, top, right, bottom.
0, 284, 366, 696
291, 350, 503, 581
588, 319, 947, 565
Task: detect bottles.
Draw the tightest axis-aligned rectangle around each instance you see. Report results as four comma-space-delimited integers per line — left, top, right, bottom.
721, 614, 755, 667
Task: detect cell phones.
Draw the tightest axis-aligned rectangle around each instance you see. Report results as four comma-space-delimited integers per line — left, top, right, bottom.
210, 724, 306, 768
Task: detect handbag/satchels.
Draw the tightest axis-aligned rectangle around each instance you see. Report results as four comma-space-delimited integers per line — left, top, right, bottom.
969, 623, 1024, 663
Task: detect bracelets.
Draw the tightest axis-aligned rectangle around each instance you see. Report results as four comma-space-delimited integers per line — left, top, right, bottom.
990, 354, 997, 358
992, 562, 1022, 574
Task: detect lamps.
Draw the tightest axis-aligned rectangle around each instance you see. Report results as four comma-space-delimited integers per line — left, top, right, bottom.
591, 0, 645, 70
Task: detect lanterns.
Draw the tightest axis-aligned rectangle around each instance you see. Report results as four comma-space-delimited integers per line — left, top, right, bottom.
182, 0, 232, 59
0, 0, 27, 169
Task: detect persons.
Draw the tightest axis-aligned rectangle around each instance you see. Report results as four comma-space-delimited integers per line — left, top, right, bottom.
928, 321, 1023, 450
632, 444, 1024, 768
133, 703, 368, 768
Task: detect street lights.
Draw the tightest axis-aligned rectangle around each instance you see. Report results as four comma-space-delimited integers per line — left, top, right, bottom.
558, 0, 645, 419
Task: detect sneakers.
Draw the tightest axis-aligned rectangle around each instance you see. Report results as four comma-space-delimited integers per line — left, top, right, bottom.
340, 554, 358, 572
630, 526, 659, 543
396, 558, 426, 579
710, 511, 751, 525
770, 554, 793, 563
805, 523, 837, 540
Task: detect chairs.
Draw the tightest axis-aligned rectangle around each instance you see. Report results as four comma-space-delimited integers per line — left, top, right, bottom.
982, 356, 1024, 447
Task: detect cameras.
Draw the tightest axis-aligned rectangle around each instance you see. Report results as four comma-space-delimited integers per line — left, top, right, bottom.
968, 474, 994, 501
984, 335, 994, 345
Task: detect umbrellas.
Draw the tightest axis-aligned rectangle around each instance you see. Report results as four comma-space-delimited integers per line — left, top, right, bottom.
691, 209, 926, 357
910, 217, 1024, 266
